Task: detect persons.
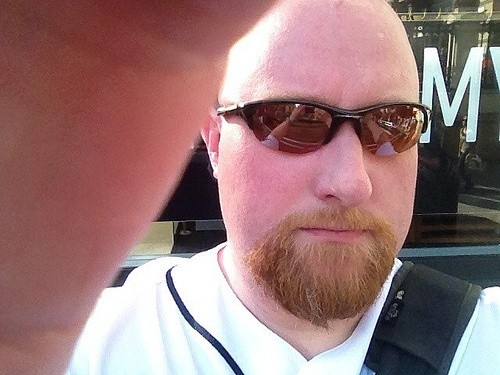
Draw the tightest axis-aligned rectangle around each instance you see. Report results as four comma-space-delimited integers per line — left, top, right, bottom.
0, 0, 500, 375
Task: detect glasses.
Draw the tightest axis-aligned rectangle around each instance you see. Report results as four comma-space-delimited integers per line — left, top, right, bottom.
216, 96, 433, 156
